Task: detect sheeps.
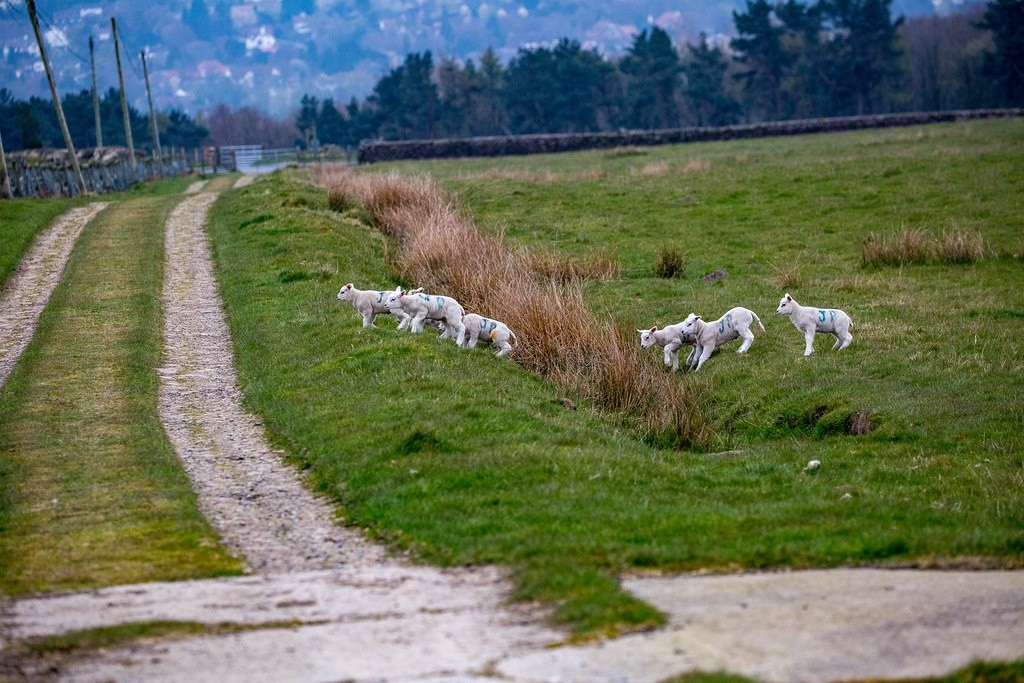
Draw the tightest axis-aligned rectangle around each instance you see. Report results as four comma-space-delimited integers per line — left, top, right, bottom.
337, 283, 517, 358
637, 306, 765, 374
777, 292, 854, 357
